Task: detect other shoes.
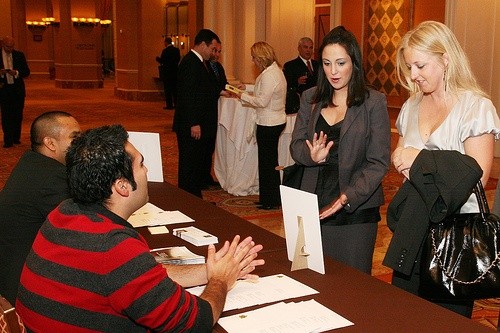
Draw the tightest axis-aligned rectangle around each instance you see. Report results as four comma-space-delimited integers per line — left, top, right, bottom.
164, 106, 174, 110
3, 144, 13, 148
15, 141, 20, 144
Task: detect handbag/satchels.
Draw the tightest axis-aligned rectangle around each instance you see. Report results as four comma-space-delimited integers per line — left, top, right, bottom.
275, 163, 304, 190
419, 179, 500, 301
285, 87, 300, 115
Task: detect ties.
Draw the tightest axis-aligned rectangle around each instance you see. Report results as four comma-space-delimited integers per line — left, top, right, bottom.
203, 60, 208, 72
212, 64, 217, 73
306, 61, 313, 75
7, 54, 13, 83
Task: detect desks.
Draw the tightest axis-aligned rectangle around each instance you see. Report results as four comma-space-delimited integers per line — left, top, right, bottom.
94, 180, 500, 333
215, 96, 297, 195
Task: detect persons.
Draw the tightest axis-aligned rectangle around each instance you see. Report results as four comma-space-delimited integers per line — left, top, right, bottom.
172, 29, 233, 206
16, 125, 265, 333
1, 110, 83, 309
156, 38, 181, 110
289, 28, 390, 276
282, 37, 320, 114
236, 42, 287, 211
382, 20, 500, 318
0, 35, 31, 148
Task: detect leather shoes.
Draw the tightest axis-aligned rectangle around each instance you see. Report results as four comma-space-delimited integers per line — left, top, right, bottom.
255, 202, 279, 209
205, 182, 221, 190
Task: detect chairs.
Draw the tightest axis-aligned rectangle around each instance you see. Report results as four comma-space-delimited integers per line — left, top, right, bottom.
0, 298, 26, 333
